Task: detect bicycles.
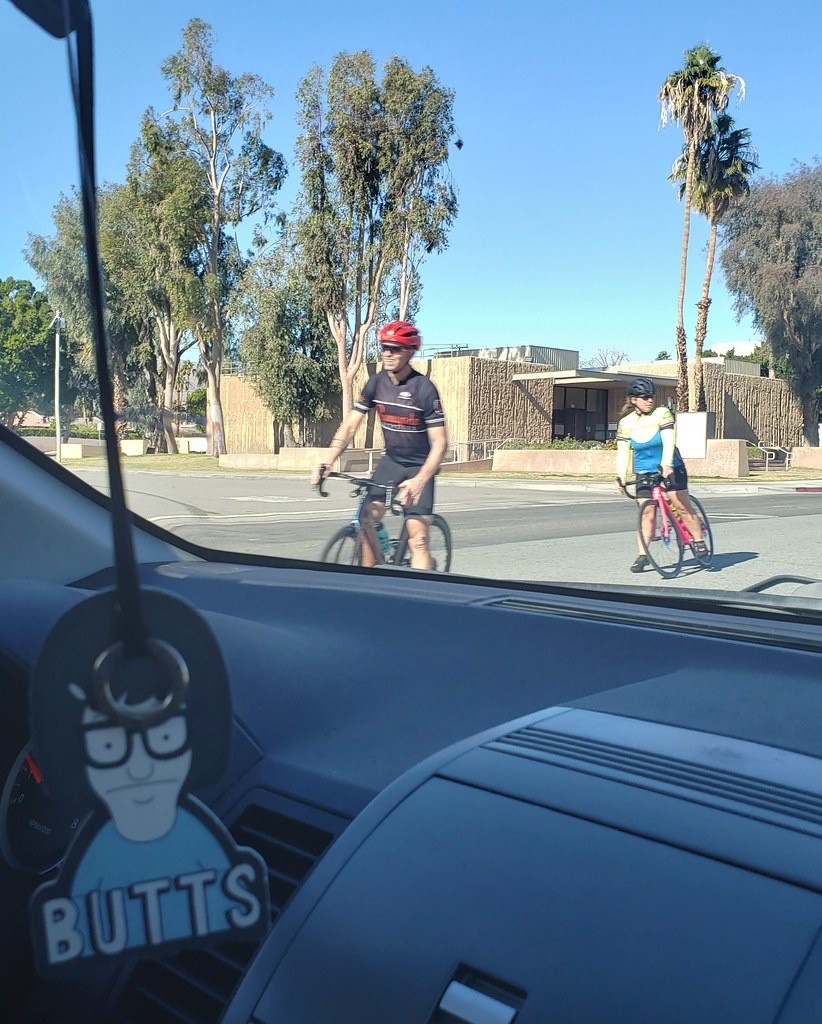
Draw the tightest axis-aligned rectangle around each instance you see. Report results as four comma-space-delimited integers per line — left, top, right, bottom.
316, 470, 453, 576
617, 463, 714, 579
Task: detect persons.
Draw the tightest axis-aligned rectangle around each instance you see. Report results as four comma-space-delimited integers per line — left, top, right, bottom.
615, 378, 708, 572
311, 320, 447, 573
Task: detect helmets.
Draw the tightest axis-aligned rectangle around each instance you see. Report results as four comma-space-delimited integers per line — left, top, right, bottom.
378, 320, 421, 350
625, 377, 657, 395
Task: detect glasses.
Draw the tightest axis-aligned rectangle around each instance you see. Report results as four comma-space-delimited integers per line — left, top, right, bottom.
380, 345, 412, 353
632, 394, 657, 400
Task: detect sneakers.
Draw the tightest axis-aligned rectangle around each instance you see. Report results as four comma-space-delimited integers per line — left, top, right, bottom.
693, 538, 708, 558
630, 555, 650, 572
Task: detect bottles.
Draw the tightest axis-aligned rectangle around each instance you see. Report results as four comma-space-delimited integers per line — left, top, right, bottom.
374, 520, 391, 553
667, 500, 680, 521
